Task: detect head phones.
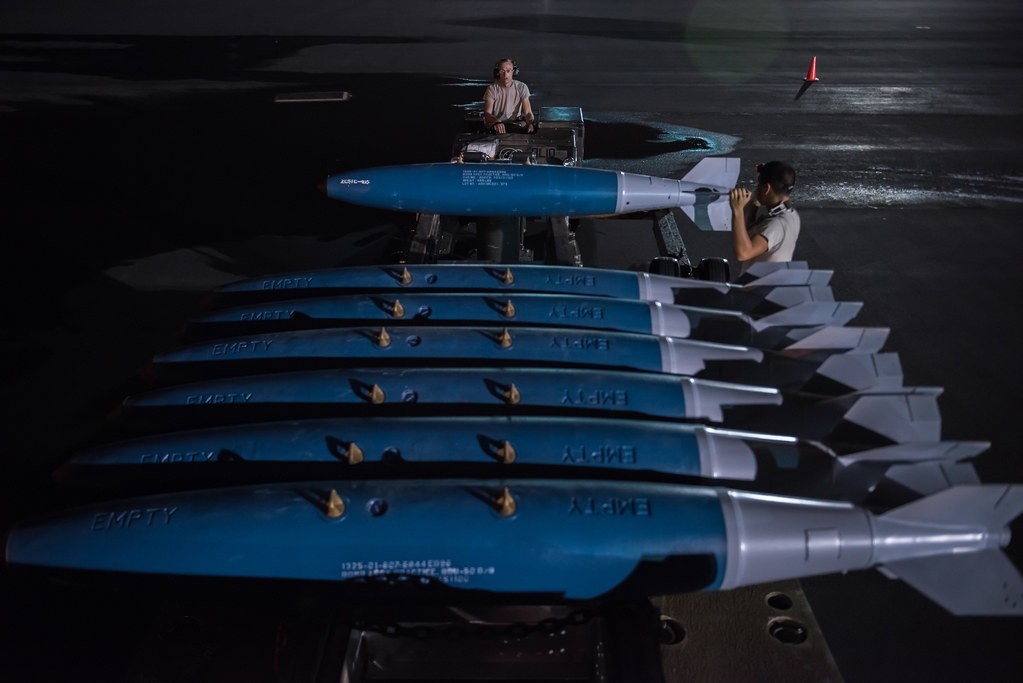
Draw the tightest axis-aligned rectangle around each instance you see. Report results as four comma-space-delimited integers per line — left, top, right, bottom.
493, 57, 519, 79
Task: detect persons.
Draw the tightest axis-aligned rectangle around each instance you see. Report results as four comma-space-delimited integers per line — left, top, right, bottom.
481, 57, 535, 134
693, 160, 802, 414
